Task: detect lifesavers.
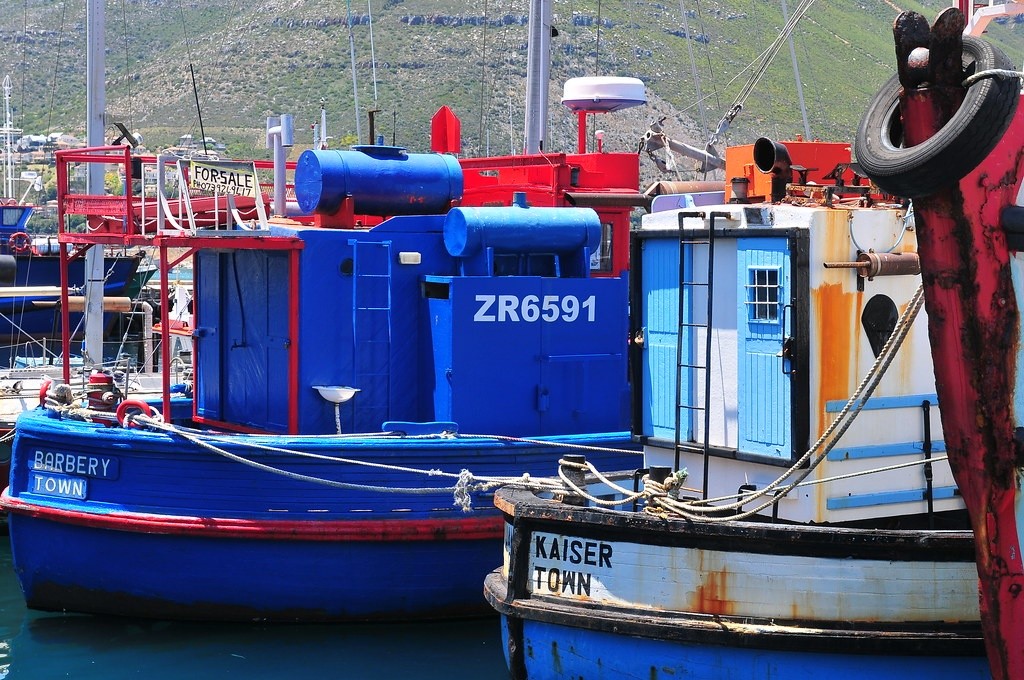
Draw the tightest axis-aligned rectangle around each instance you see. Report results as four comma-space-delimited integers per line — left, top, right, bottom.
9, 232, 31, 253
116, 399, 152, 428
40, 380, 52, 407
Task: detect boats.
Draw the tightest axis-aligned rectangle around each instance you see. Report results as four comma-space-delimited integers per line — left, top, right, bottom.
475, 3, 1023, 680
0, 3, 333, 460
0, 4, 752, 629
